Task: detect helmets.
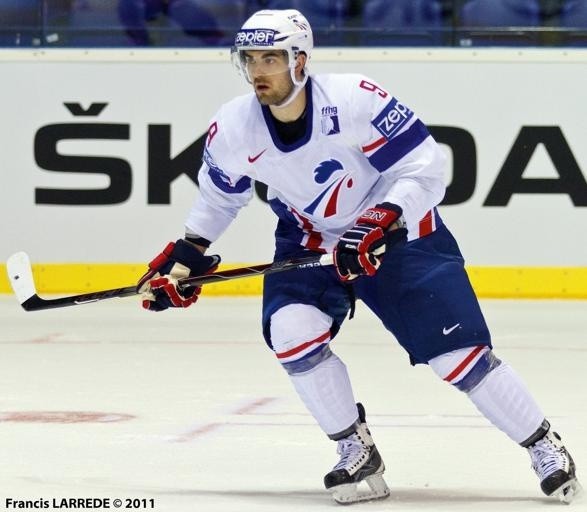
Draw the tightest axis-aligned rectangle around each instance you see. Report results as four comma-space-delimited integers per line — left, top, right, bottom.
230, 8, 313, 109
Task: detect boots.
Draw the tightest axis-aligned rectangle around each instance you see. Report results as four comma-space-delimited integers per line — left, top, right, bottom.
525, 421, 577, 497
324, 402, 386, 489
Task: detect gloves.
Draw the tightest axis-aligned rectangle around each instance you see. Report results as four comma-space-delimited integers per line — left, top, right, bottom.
332, 201, 404, 286
136, 239, 221, 313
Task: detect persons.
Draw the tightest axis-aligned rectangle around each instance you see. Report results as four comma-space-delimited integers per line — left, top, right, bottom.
135, 9, 576, 497
